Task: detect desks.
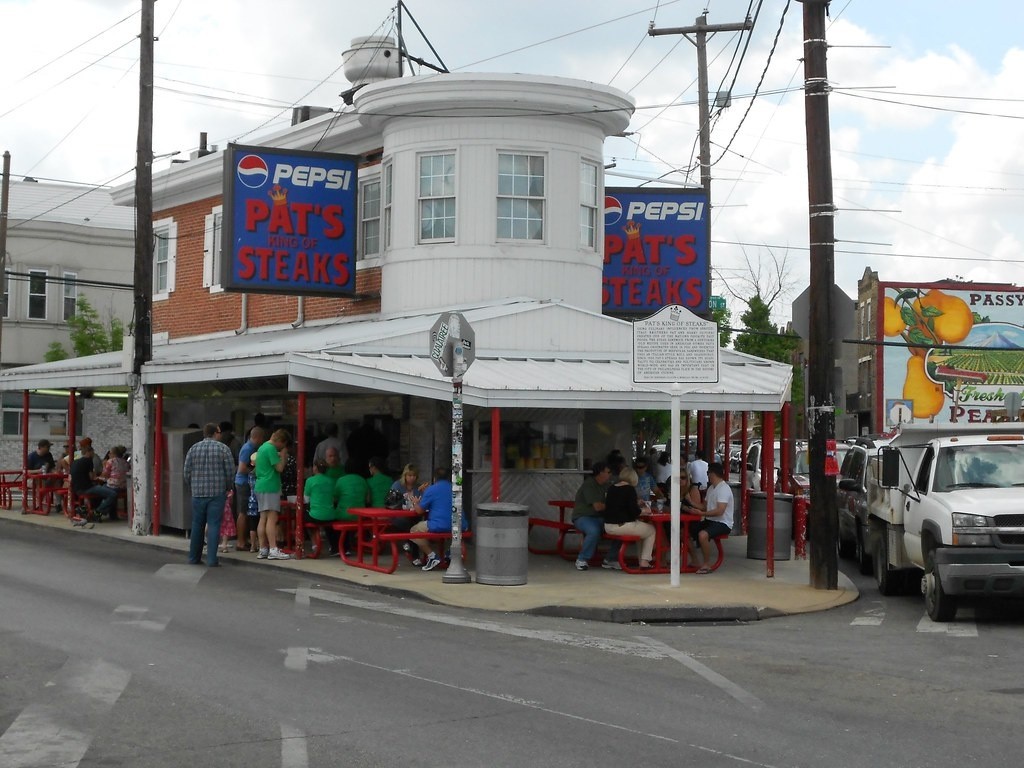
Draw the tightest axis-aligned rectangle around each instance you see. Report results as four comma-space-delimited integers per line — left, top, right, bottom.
278, 500, 309, 556
0, 470, 24, 510
346, 508, 427, 573
544, 504, 703, 572
26, 473, 66, 513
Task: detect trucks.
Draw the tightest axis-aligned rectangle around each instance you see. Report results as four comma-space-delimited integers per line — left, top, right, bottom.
865, 421, 1024, 622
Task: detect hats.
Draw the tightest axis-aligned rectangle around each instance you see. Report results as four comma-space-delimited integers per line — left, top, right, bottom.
37, 439, 53, 448
79, 438, 93, 448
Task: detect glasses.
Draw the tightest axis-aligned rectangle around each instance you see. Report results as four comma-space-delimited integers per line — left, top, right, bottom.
680, 475, 686, 480
706, 471, 712, 476
634, 464, 646, 470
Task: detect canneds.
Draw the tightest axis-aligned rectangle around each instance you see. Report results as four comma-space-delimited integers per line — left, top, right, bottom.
42, 464, 47, 475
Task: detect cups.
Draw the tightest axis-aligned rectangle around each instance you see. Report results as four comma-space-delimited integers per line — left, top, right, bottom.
657, 499, 663, 514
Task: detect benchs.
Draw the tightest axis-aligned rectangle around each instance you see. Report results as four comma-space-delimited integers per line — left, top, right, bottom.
0, 483, 470, 574
655, 532, 731, 569
531, 517, 655, 574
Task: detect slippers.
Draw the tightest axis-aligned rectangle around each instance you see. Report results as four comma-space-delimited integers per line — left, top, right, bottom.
695, 568, 713, 575
641, 564, 654, 570
687, 562, 700, 569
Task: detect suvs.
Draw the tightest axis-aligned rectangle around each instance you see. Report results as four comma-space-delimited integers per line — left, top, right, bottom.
774, 440, 850, 539
839, 438, 893, 575
743, 440, 782, 488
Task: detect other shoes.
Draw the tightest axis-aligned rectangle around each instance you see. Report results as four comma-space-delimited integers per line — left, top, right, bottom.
250, 546, 259, 554
208, 562, 219, 567
236, 543, 252, 552
189, 559, 204, 565
413, 558, 422, 565
91, 511, 102, 523
202, 544, 208, 552
55, 504, 64, 513
75, 507, 87, 516
216, 547, 228, 553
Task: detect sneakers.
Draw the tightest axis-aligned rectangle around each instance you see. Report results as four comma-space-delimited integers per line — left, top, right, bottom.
443, 552, 464, 562
602, 559, 623, 569
575, 559, 589, 571
422, 554, 440, 571
267, 548, 290, 560
256, 547, 268, 559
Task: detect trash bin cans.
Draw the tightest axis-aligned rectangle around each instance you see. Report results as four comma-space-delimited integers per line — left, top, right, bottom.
747, 492, 792, 560
727, 480, 744, 534
475, 502, 531, 585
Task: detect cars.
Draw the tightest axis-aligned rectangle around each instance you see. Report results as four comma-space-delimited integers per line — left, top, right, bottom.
678, 435, 721, 463
651, 443, 666, 454
717, 442, 741, 474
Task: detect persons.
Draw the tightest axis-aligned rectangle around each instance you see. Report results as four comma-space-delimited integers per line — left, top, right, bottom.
28, 436, 127, 523
186, 413, 468, 572
574, 445, 736, 578
